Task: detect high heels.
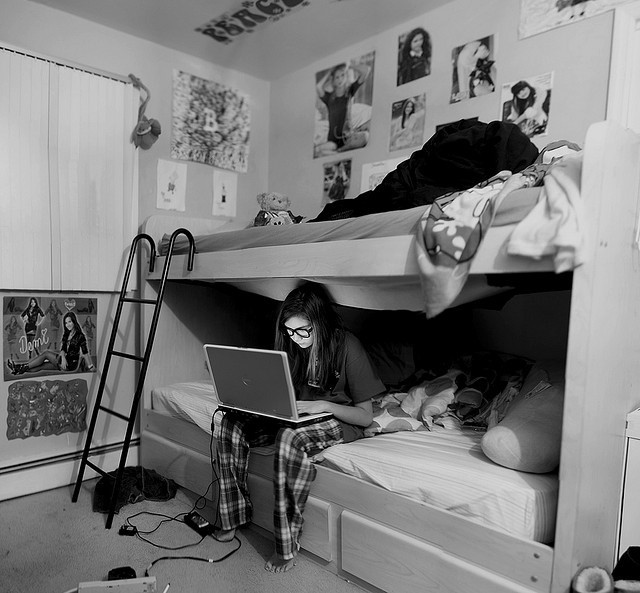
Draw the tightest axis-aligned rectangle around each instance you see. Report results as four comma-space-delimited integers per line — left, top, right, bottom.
12, 361, 30, 375
8, 359, 13, 371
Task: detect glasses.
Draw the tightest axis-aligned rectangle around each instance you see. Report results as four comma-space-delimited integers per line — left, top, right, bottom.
278, 326, 313, 338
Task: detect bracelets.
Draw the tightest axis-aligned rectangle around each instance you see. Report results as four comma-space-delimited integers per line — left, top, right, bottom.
88, 365, 94, 371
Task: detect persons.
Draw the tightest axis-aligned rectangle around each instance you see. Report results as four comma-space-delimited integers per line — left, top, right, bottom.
507, 81, 549, 136
45, 299, 63, 350
76, 299, 95, 313
326, 162, 350, 200
20, 297, 44, 359
314, 60, 372, 154
456, 41, 490, 101
4, 316, 22, 361
6, 312, 96, 375
397, 27, 430, 84
5, 297, 23, 315
392, 98, 426, 149
81, 316, 95, 356
210, 281, 389, 574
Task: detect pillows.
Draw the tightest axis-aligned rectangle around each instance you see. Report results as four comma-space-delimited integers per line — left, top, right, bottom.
480, 367, 563, 473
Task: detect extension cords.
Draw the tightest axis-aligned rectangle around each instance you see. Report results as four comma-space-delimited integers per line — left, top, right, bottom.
77, 577, 158, 593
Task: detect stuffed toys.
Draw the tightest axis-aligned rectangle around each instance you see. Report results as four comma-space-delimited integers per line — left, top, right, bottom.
245, 191, 306, 228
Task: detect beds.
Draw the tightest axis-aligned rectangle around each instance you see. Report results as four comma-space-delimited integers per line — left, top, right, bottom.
138, 120, 640, 593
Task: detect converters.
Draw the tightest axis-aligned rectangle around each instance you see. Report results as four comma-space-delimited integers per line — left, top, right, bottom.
184, 511, 215, 537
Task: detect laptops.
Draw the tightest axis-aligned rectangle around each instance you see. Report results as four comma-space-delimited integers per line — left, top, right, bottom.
204, 343, 334, 430
